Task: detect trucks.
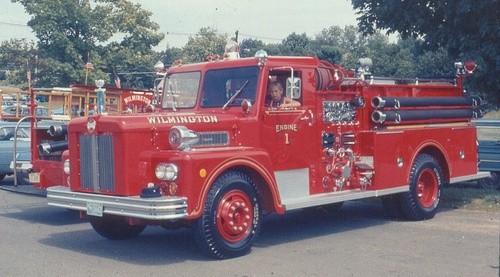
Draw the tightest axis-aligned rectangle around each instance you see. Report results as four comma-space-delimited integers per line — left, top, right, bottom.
42, 50, 492, 261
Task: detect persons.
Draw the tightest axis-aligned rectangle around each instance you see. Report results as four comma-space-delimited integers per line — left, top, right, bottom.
269, 83, 301, 108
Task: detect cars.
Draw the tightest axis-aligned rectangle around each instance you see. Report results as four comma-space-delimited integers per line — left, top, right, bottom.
470, 118, 500, 189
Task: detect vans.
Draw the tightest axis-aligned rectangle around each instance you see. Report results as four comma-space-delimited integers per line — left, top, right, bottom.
0, 122, 34, 185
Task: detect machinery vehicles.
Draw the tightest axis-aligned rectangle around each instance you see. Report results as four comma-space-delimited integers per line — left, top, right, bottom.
14, 61, 199, 190
